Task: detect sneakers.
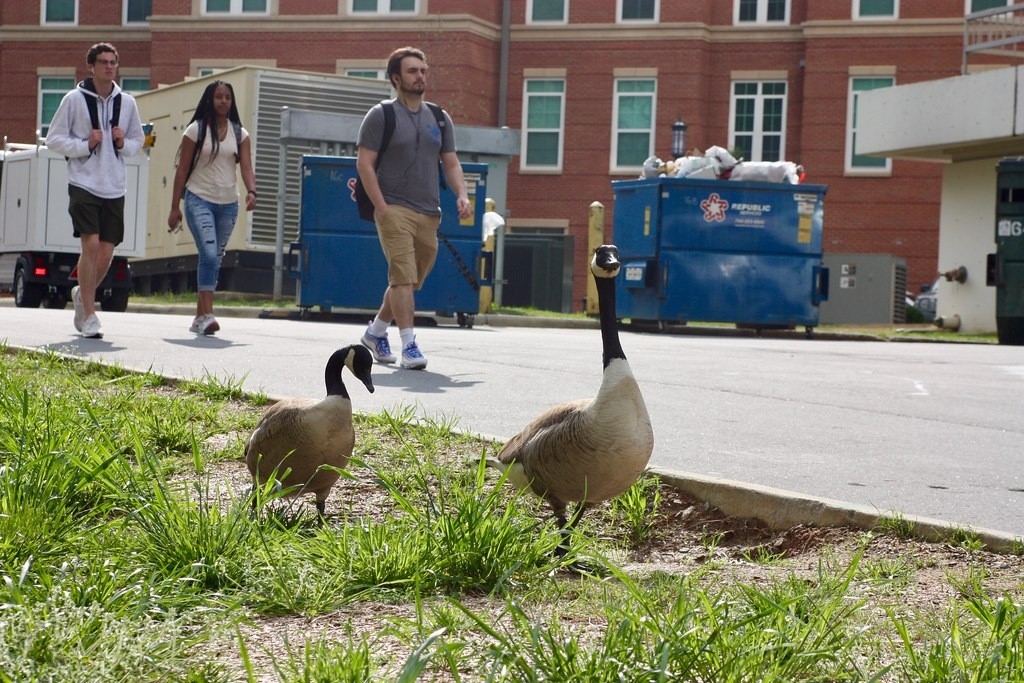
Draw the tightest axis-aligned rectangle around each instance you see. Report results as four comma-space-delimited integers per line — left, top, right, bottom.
71, 286, 85, 333
82, 314, 105, 340
188, 317, 215, 336
360, 320, 396, 364
199, 315, 220, 336
399, 334, 428, 369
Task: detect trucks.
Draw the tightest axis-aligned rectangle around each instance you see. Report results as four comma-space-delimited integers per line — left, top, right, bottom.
1, 130, 151, 312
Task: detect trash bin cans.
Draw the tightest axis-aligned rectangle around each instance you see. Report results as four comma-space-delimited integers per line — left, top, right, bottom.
288, 154, 488, 328
995, 156, 1024, 345
611, 178, 829, 337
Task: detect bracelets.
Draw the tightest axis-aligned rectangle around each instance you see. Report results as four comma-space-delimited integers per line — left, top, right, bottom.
248, 191, 256, 197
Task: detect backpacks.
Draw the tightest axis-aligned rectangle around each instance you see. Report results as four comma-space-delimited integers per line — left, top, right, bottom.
355, 100, 446, 221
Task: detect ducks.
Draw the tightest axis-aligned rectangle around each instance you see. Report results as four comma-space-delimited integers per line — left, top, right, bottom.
243, 345, 375, 525
473, 246, 654, 574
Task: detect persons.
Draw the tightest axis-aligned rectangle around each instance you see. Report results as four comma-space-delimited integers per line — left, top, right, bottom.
168, 81, 257, 335
46, 43, 144, 338
356, 47, 474, 368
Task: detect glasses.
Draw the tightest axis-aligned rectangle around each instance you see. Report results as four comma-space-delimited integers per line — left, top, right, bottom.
92, 59, 117, 66
